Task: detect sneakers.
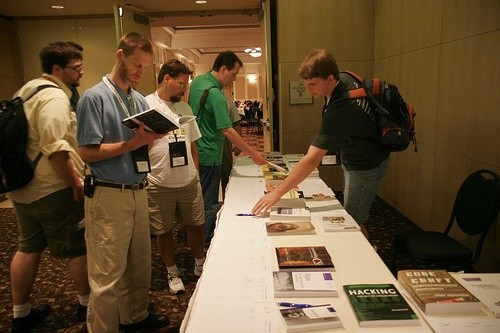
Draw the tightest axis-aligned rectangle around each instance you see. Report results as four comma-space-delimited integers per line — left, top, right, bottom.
167, 270, 186, 294
194, 263, 204, 275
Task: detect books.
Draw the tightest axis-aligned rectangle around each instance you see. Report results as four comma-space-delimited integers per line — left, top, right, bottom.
261, 152, 500, 333
122, 108, 195, 134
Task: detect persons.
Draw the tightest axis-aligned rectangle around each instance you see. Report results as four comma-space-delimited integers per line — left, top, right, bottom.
5, 32, 267, 333
251, 49, 389, 240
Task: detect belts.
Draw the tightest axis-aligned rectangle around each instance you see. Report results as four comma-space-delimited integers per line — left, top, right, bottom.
96, 178, 150, 191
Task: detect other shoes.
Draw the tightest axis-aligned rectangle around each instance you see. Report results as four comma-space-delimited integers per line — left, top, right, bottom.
10, 303, 51, 333
76, 302, 87, 321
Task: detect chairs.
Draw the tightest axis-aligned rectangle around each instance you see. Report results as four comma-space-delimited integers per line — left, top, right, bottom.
392, 169, 500, 276
237, 108, 263, 132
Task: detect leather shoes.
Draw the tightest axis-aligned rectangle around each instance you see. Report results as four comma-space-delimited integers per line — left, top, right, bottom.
122, 313, 170, 332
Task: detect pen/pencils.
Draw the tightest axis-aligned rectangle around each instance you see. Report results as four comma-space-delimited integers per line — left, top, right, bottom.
237, 214, 255, 216
278, 302, 310, 307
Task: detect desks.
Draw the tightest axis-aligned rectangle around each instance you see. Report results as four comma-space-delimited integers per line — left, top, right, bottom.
178, 153, 500, 333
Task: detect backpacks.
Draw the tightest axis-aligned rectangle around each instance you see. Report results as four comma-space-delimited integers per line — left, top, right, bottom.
0, 84, 62, 194
322, 71, 419, 154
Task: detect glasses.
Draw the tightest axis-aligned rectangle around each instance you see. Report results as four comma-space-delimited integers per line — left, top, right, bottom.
173, 76, 190, 91
65, 64, 85, 72
